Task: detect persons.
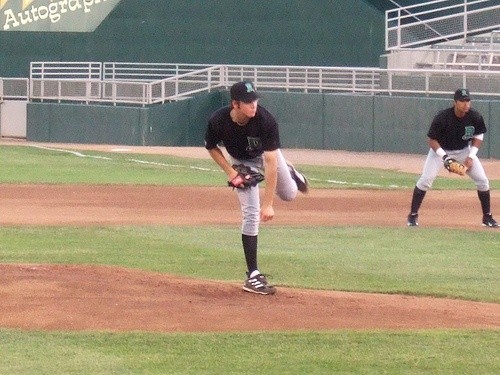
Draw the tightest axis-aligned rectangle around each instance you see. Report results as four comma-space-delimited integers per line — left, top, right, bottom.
407, 89, 500, 228
204, 80, 310, 295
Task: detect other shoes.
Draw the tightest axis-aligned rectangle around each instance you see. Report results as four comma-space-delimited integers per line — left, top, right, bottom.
407, 213, 419, 226
482, 213, 500, 227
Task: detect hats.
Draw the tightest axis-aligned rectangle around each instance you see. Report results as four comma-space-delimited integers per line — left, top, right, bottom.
230, 81, 258, 103
453, 89, 471, 101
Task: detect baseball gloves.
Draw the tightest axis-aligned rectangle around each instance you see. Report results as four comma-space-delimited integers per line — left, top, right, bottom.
444, 158, 469, 177
226, 163, 264, 190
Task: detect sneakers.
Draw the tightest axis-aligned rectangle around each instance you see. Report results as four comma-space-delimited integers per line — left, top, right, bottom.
285, 160, 309, 193
242, 273, 277, 296
